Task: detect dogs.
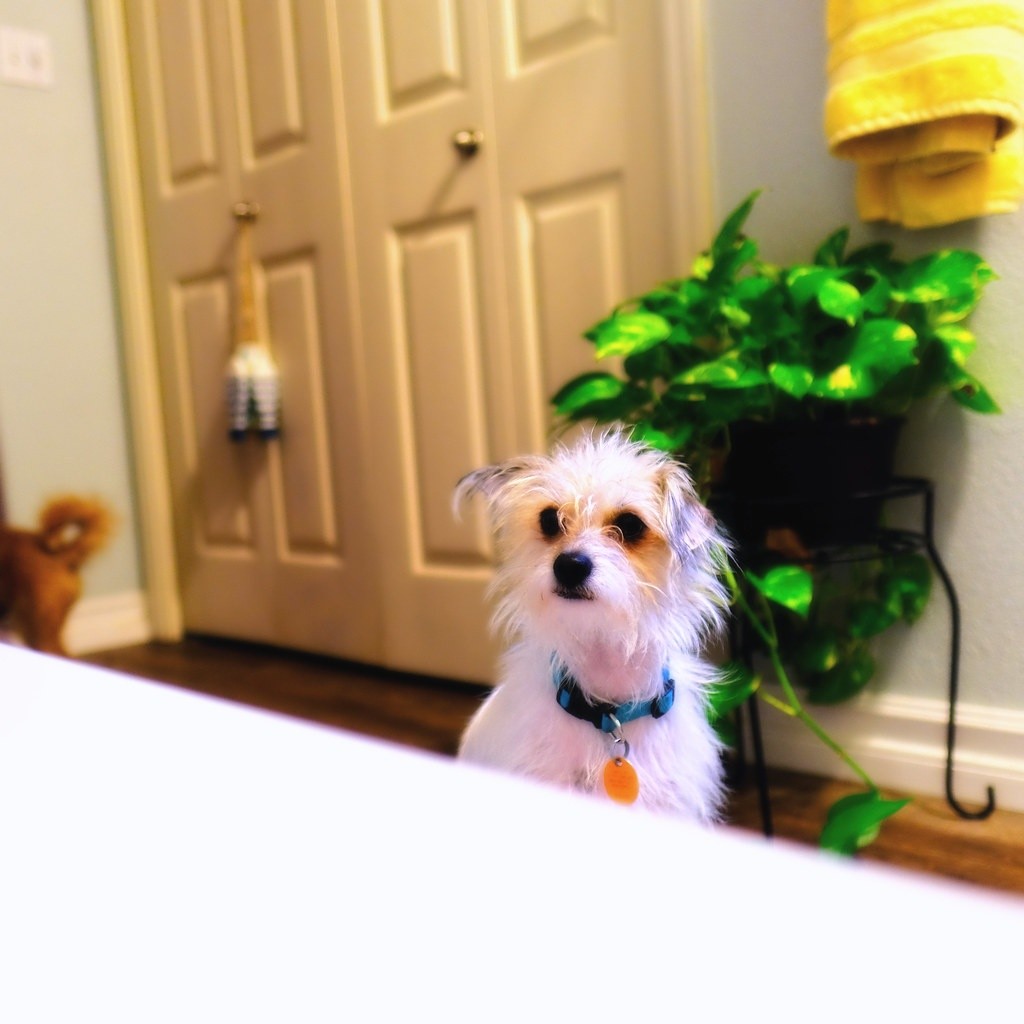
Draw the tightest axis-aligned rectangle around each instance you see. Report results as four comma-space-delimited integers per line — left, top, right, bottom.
1, 491, 119, 660
449, 420, 743, 827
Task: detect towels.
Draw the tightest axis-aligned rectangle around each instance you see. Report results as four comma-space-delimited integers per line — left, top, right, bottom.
821, 1, 1024, 230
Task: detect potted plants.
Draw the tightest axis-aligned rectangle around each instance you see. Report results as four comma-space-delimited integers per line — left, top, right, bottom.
548, 186, 1005, 855
